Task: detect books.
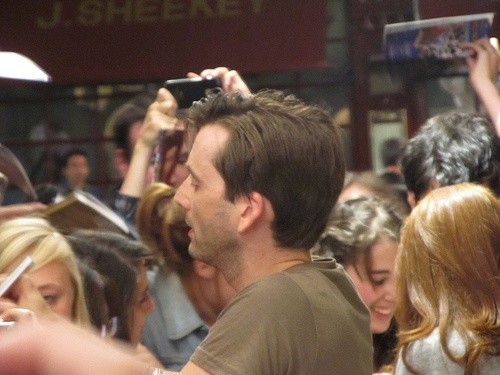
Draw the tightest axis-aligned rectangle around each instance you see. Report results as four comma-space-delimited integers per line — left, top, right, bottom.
383, 12, 495, 63
42, 191, 136, 240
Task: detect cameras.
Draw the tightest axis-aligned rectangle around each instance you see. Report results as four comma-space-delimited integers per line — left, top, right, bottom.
164, 77, 222, 108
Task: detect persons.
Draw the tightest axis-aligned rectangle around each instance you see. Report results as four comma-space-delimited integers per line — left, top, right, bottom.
311, 197, 407, 375
457, 36, 500, 137
59, 150, 107, 202
0, 216, 94, 334
0, 89, 373, 375
387, 182, 500, 375
135, 185, 250, 372
401, 111, 500, 211
0, 174, 49, 220
65, 227, 154, 349
378, 138, 404, 184
104, 96, 155, 188
113, 67, 254, 269
